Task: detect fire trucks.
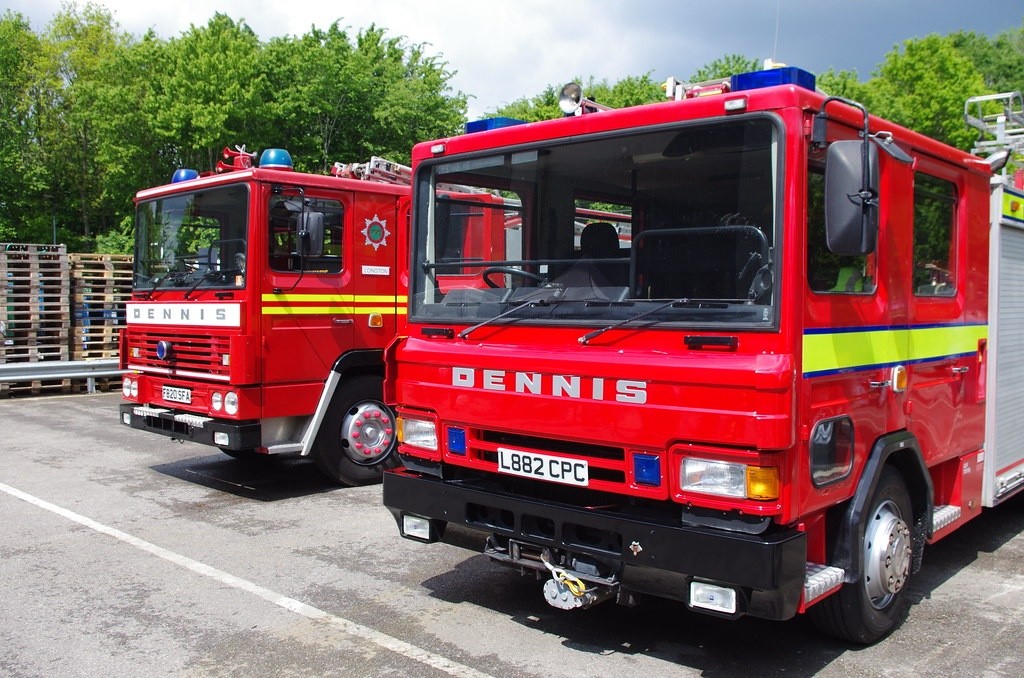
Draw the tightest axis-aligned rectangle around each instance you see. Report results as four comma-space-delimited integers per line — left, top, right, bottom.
381, 57, 1024, 646
119, 141, 634, 487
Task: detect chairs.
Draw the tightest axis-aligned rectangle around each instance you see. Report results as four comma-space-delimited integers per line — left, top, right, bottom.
581, 222, 620, 257
